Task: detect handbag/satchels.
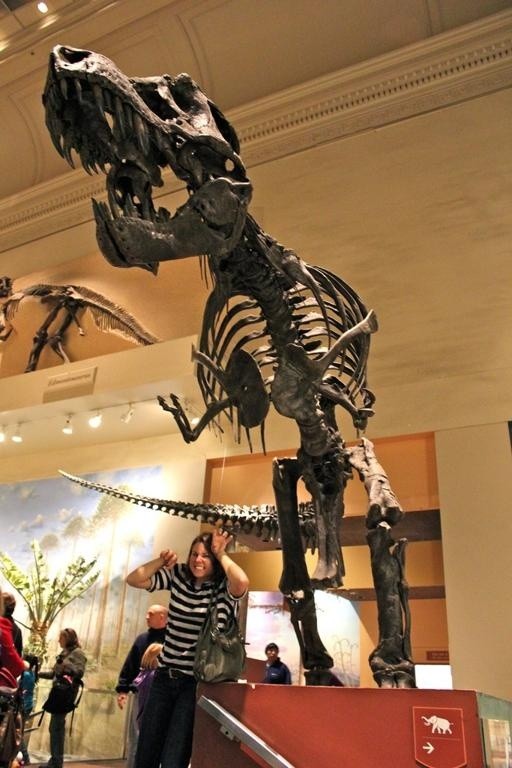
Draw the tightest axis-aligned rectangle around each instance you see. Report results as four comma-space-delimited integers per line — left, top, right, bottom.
193, 577, 243, 683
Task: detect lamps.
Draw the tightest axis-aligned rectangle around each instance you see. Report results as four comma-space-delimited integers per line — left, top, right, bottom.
11, 420, 22, 442
186, 408, 200, 425
62, 415, 74, 434
122, 403, 136, 423
88, 409, 104, 428
0, 423, 9, 442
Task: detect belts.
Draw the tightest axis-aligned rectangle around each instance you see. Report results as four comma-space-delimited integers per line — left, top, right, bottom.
158, 667, 192, 680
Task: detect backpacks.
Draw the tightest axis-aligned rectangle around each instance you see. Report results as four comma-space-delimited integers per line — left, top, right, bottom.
42, 676, 83, 714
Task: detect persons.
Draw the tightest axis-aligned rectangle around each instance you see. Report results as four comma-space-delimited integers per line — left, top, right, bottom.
37, 628, 87, 768
113, 604, 168, 768
3, 591, 23, 661
0, 586, 36, 768
134, 644, 164, 735
127, 528, 250, 768
17, 652, 39, 765
262, 642, 289, 684
